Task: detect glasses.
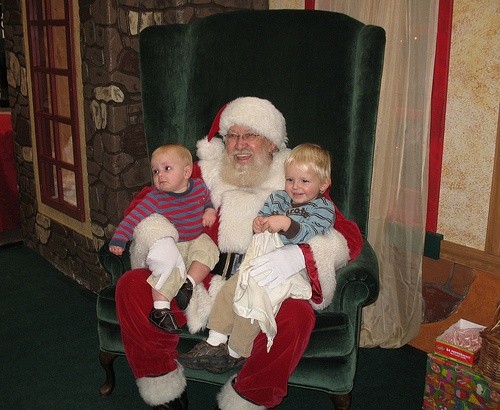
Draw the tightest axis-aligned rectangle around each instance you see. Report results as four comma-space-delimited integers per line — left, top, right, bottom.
226, 132, 259, 142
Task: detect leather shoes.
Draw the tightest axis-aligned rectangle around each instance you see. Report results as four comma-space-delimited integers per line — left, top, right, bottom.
205, 354, 245, 374
178, 340, 228, 369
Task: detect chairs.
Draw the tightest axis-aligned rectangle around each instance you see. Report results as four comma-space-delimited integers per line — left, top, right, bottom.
97, 9, 386, 410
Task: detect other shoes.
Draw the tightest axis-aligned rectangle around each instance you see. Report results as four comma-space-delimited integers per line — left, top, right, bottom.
148, 307, 183, 334
176, 278, 193, 310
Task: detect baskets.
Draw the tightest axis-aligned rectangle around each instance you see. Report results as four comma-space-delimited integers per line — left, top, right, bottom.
478, 301, 500, 391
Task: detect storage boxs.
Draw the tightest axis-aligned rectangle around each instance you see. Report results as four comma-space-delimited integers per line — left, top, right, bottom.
435, 319, 487, 367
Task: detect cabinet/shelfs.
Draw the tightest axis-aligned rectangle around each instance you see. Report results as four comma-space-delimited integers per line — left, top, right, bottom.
423, 352, 500, 410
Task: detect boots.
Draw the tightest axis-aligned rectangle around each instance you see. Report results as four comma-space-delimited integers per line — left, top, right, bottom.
216, 374, 265, 410
135, 358, 188, 410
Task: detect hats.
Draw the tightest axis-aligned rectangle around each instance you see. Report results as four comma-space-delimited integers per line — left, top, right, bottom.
197, 96, 287, 160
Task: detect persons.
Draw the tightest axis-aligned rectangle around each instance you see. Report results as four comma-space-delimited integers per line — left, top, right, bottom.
177, 144, 336, 374
108, 96, 364, 410
109, 145, 216, 335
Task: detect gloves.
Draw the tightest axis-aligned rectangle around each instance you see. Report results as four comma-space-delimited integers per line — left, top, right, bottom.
249, 244, 306, 289
145, 236, 187, 291
